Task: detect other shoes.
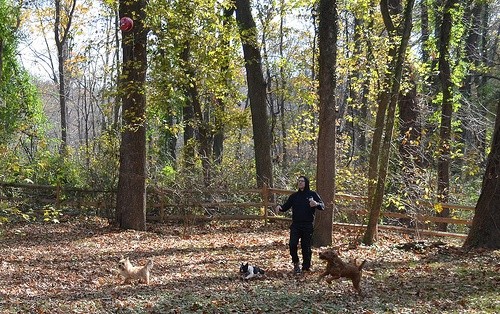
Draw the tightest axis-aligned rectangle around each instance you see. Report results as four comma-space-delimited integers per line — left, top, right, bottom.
294, 262, 300, 272
303, 269, 309, 273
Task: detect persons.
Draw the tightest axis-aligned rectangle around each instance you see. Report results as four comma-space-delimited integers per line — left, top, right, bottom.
275, 176, 325, 275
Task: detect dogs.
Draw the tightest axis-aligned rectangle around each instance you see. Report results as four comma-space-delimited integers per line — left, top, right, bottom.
316, 250, 367, 298
118, 255, 153, 286
240, 262, 265, 280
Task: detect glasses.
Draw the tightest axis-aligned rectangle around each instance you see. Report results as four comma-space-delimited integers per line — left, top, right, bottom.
298, 180, 305, 182
